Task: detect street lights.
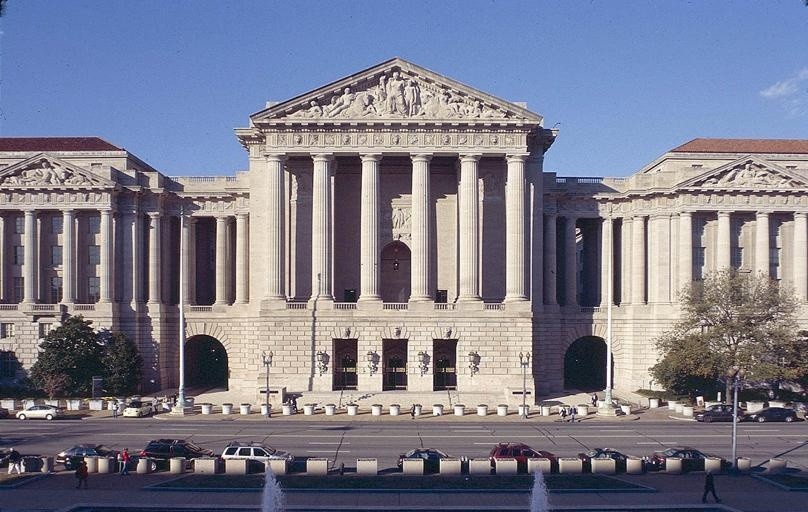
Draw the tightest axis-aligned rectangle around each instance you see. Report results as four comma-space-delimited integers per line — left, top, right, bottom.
519, 352, 530, 419
262, 351, 273, 418
727, 366, 746, 467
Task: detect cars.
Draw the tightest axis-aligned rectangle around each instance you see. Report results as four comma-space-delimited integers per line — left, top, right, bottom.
0, 408, 9, 417
56, 439, 296, 471
15, 405, 65, 420
490, 442, 557, 472
578, 448, 709, 473
750, 407, 797, 423
0, 448, 19, 464
693, 405, 745, 423
122, 402, 152, 417
397, 448, 468, 473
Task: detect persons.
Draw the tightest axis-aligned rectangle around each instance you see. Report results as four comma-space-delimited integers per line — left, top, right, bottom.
75, 462, 88, 489
701, 164, 794, 188
559, 407, 566, 422
592, 393, 598, 407
152, 396, 159, 414
702, 470, 722, 503
569, 405, 577, 423
171, 393, 177, 406
121, 447, 134, 475
291, 394, 297, 413
112, 402, 117, 419
286, 71, 506, 118
1, 162, 92, 185
6, 448, 21, 474
